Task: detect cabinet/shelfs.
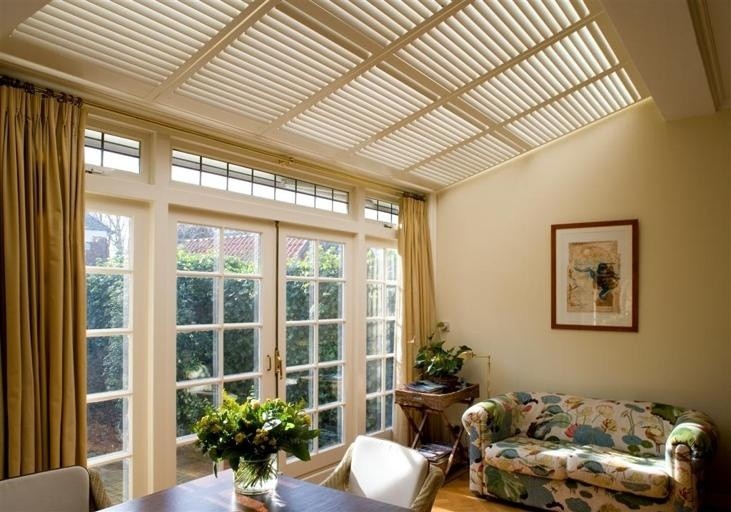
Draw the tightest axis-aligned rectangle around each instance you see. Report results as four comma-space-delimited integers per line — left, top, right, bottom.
394, 383, 479, 487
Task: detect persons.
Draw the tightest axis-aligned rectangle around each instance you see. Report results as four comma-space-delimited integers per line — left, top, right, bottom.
593, 263, 618, 306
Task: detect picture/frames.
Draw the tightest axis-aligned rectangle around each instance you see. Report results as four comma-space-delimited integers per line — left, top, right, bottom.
551, 220, 639, 332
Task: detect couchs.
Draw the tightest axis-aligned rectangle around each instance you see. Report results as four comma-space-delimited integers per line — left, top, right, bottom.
462, 391, 720, 512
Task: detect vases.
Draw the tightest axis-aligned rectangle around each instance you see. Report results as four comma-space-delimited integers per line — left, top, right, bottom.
234, 459, 277, 495
428, 375, 459, 389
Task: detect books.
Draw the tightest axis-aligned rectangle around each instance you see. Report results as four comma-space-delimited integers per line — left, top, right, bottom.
406, 380, 445, 393
418, 443, 452, 461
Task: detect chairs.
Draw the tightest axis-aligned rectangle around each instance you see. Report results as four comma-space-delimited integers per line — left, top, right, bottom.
0, 465, 111, 512
317, 435, 444, 511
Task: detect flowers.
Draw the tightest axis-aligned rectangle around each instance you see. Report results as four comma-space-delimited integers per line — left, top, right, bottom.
410, 321, 475, 375
193, 388, 327, 488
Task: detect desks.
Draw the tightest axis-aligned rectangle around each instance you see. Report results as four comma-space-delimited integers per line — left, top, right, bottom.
95, 467, 420, 512
